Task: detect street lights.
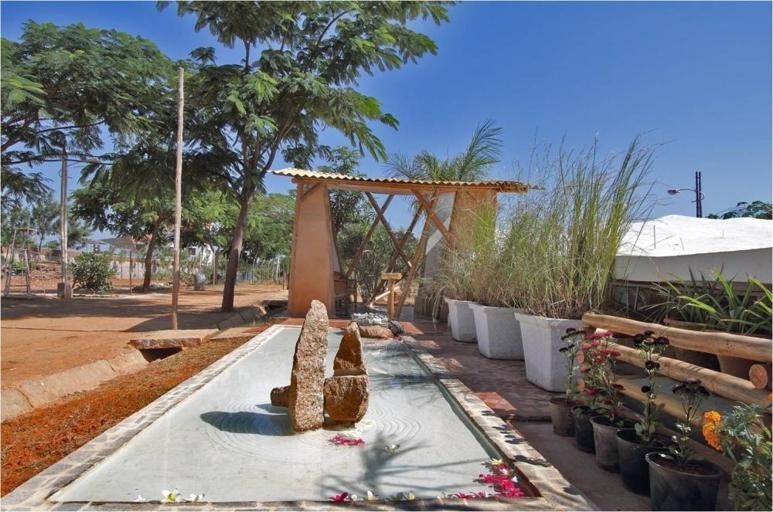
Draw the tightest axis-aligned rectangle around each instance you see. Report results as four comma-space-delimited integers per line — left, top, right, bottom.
49, 131, 71, 299
667, 171, 704, 218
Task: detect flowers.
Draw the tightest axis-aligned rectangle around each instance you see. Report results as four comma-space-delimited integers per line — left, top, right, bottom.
659, 380, 708, 470
633, 331, 669, 443
579, 330, 626, 416
583, 350, 625, 427
558, 327, 586, 400
703, 394, 773, 512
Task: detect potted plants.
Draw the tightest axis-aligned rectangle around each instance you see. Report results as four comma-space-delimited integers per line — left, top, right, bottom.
679, 271, 772, 382
437, 203, 502, 342
648, 268, 732, 367
515, 141, 659, 394
471, 133, 565, 358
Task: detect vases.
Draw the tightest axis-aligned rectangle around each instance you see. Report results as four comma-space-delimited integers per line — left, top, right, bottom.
590, 416, 638, 472
551, 398, 584, 440
573, 405, 612, 453
615, 430, 669, 495
647, 452, 722, 511
